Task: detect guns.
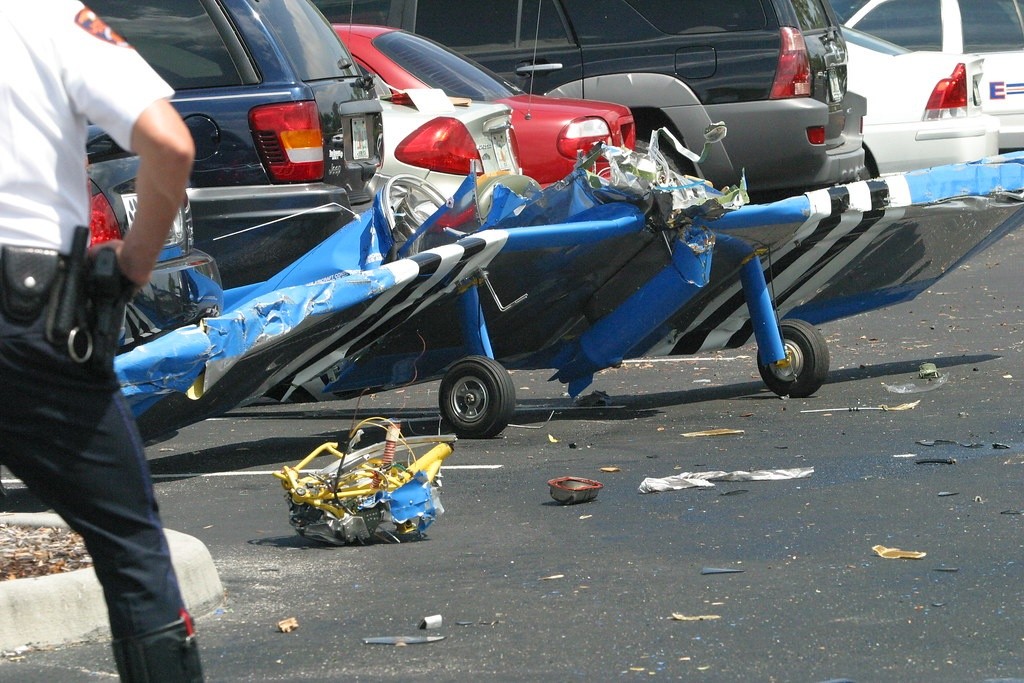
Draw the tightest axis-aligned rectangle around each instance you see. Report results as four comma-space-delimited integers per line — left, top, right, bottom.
96, 246, 130, 383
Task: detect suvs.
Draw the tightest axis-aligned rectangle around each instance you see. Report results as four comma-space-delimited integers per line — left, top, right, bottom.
78, 1, 392, 287
314, 0, 870, 200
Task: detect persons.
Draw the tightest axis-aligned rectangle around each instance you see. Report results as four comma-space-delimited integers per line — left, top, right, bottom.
0, 0, 206, 683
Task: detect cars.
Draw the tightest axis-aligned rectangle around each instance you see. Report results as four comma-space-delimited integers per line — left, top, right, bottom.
326, 22, 641, 204
838, 0, 1024, 151
836, 22, 1002, 178
337, 72, 536, 250
